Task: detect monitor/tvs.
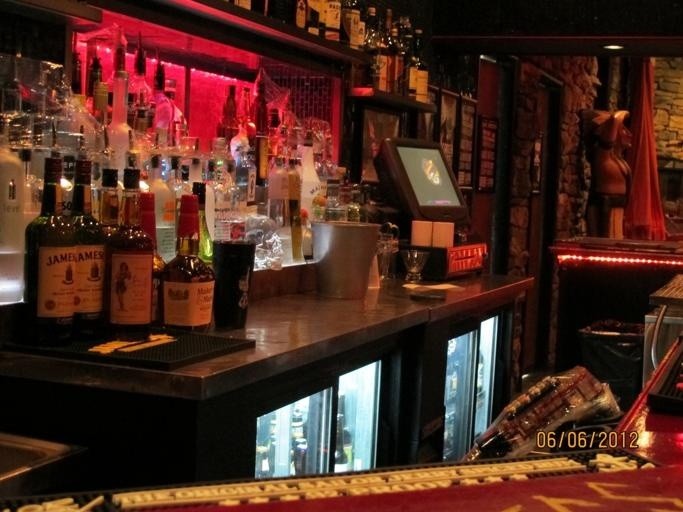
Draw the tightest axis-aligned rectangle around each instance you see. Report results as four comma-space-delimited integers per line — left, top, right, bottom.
373, 137, 469, 223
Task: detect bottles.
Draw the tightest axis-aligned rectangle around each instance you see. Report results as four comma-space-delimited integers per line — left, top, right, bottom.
23, 156, 72, 346
158, 192, 216, 335
105, 167, 155, 342
192, 180, 215, 275
67, 155, 106, 341
87, 0, 430, 262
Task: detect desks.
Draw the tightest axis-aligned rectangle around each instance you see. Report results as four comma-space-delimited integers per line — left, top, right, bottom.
547, 244, 683, 365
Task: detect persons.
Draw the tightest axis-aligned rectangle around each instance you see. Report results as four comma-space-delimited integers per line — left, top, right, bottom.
585, 109, 633, 240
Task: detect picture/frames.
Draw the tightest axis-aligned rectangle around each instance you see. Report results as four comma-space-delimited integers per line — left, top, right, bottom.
348, 81, 480, 195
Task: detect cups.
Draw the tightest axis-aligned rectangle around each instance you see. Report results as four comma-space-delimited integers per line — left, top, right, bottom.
401, 249, 431, 283
212, 239, 257, 331
